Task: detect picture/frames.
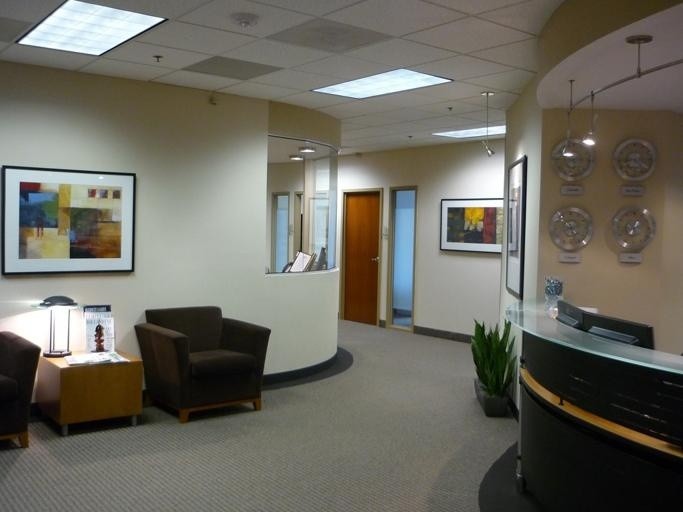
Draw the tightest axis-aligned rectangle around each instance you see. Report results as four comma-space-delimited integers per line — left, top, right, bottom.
504, 154, 525, 301
507, 199, 518, 252
0, 162, 136, 277
438, 197, 507, 253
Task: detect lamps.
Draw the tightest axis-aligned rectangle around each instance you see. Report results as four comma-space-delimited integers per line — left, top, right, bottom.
557, 31, 681, 160
287, 141, 315, 163
34, 295, 80, 357
480, 89, 500, 157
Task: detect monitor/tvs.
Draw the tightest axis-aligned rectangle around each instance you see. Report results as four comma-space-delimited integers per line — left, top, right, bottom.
583, 311, 654, 350
556, 300, 584, 331
303, 253, 318, 272
316, 247, 328, 270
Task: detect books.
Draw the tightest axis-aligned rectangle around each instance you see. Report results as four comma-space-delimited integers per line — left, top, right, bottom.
64, 304, 131, 366
289, 250, 316, 272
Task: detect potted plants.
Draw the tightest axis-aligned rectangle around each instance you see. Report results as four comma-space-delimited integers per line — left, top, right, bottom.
459, 314, 521, 418
543, 274, 562, 318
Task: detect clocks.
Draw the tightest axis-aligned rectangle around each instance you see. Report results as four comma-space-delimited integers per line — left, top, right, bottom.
610, 207, 654, 255
545, 207, 594, 252
611, 136, 657, 181
548, 137, 595, 182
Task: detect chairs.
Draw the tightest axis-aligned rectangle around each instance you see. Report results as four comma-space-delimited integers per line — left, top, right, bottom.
0, 330, 41, 449
131, 304, 271, 425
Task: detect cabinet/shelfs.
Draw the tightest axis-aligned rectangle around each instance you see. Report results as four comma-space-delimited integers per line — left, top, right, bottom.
34, 345, 145, 437
